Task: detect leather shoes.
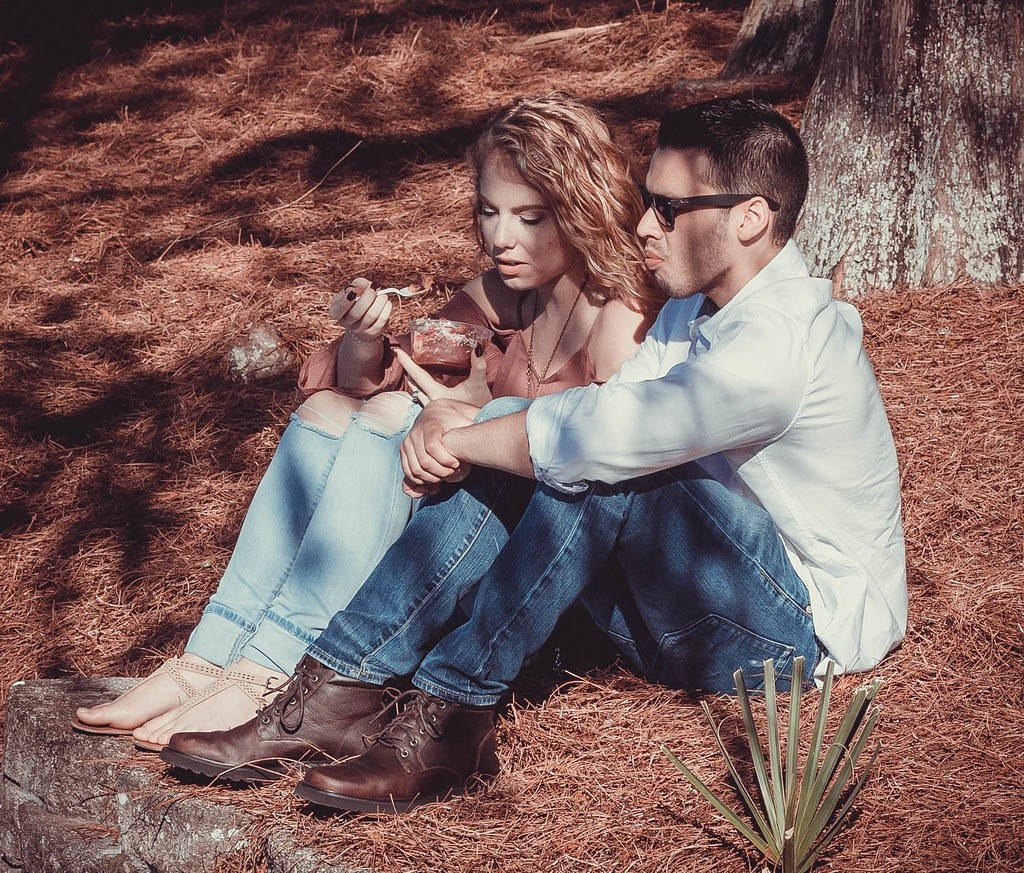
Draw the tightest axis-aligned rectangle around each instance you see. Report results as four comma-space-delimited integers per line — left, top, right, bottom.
293, 685, 500, 815
158, 652, 410, 787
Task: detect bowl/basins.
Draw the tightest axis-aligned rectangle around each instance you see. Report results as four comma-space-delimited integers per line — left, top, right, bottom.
409, 318, 494, 373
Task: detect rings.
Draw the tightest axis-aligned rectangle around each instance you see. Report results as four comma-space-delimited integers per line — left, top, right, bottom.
412, 389, 420, 402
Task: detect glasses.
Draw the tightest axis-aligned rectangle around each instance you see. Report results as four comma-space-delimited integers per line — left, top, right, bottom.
637, 184, 782, 228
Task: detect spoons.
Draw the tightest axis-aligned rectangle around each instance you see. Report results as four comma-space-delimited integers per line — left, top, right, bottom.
359, 285, 429, 300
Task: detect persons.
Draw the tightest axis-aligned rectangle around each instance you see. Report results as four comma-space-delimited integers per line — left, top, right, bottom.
163, 97, 908, 813
65, 97, 670, 752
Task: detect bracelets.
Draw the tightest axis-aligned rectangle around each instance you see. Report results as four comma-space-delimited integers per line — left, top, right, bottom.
348, 327, 389, 348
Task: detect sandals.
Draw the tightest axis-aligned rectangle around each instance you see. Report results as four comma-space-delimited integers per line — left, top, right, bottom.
70, 658, 223, 735
131, 664, 290, 751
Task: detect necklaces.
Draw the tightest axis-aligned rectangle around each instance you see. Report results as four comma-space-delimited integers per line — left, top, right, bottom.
525, 271, 589, 400
